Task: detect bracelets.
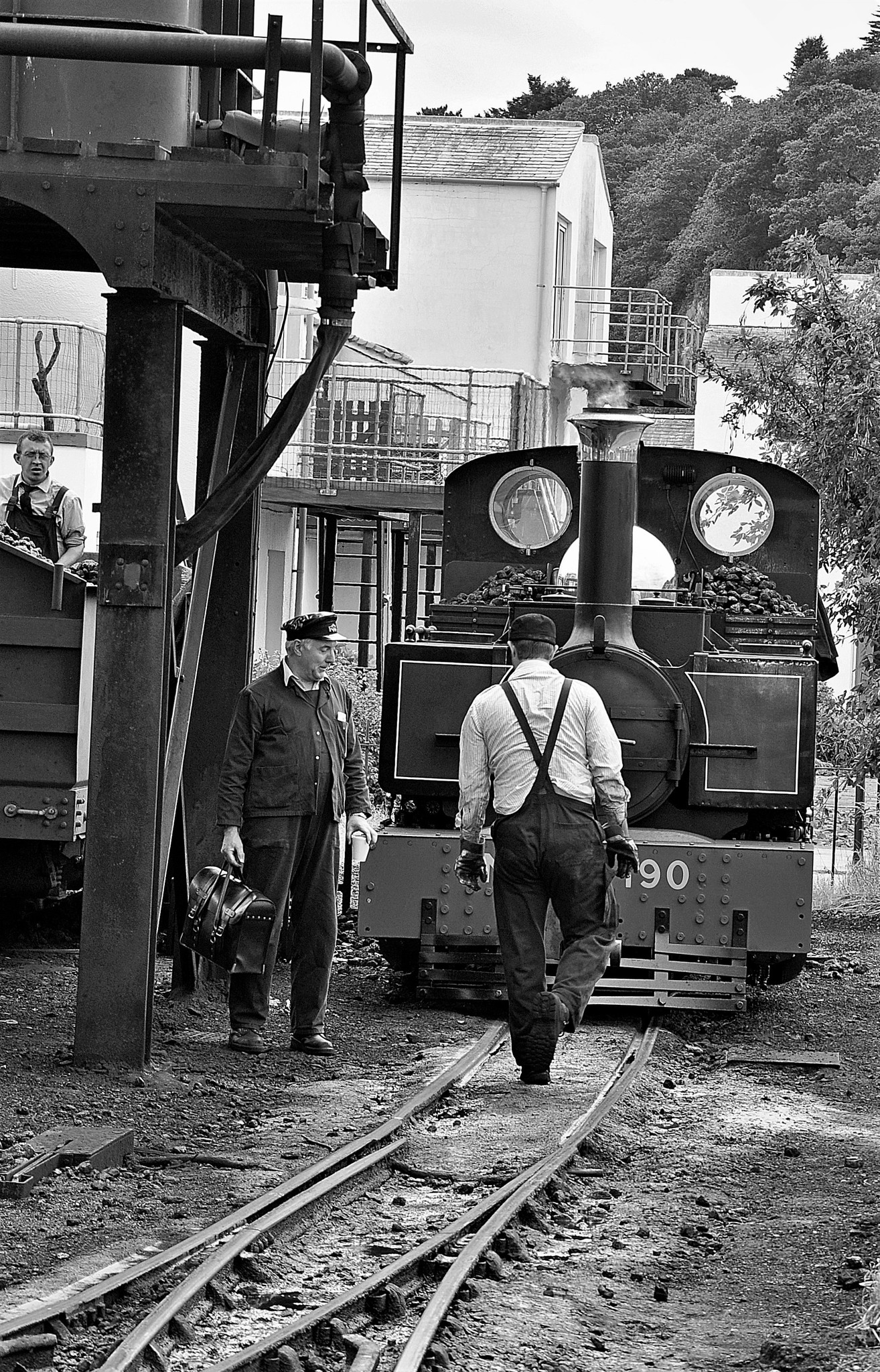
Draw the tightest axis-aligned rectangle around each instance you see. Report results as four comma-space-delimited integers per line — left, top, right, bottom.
350, 813, 367, 819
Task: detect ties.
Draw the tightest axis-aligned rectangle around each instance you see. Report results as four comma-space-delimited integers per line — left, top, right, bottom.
20, 482, 39, 515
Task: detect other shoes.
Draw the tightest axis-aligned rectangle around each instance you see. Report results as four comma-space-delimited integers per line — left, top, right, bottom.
229, 1027, 268, 1052
520, 1073, 550, 1085
526, 991, 569, 1073
290, 1033, 334, 1054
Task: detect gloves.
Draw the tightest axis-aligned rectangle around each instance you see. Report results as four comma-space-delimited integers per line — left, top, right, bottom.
602, 818, 638, 879
454, 837, 488, 891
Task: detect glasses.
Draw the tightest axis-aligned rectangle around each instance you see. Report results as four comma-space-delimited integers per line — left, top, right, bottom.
20, 453, 51, 459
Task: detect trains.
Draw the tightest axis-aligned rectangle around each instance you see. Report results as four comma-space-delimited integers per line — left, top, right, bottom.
352, 384, 841, 1018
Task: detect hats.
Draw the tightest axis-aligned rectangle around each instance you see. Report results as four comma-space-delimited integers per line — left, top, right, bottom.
280, 611, 346, 641
508, 614, 556, 647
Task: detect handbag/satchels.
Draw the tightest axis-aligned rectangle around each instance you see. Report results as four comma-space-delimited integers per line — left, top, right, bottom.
179, 850, 277, 974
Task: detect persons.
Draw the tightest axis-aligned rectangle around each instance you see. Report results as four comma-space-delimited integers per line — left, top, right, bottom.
0, 429, 87, 568
214, 611, 377, 1056
453, 613, 640, 1086
